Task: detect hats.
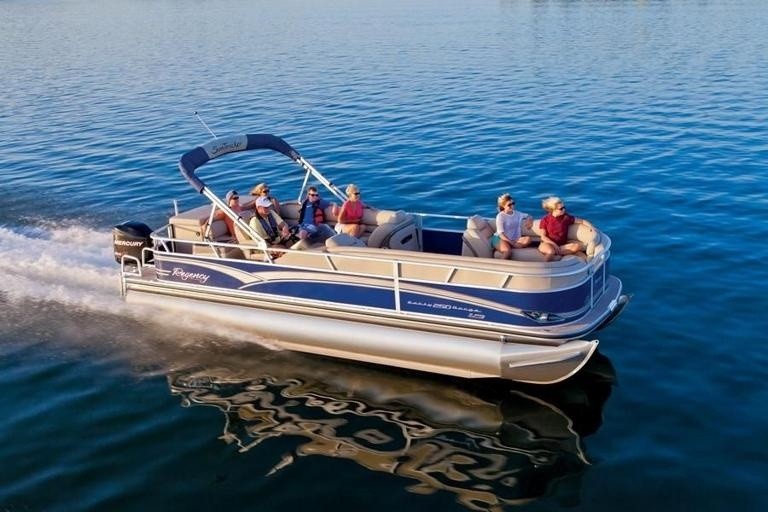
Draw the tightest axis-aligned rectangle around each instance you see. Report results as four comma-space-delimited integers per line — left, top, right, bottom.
226, 190, 237, 206
256, 195, 273, 207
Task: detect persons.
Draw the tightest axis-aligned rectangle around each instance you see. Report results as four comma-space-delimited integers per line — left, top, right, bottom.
335, 183, 380, 245
539, 196, 599, 263
242, 183, 283, 226
489, 193, 535, 260
297, 186, 337, 247
249, 196, 294, 261
204, 189, 255, 257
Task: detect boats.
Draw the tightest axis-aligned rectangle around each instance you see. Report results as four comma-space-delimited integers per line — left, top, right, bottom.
159, 338, 619, 512
112, 111, 631, 386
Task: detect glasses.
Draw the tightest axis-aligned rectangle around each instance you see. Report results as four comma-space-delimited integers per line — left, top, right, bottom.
309, 194, 319, 196
263, 189, 269, 193
353, 192, 360, 195
508, 202, 515, 206
557, 206, 565, 210
232, 197, 239, 200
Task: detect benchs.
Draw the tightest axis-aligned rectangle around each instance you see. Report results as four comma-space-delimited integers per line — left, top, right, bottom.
202, 202, 407, 260
460, 214, 601, 263
